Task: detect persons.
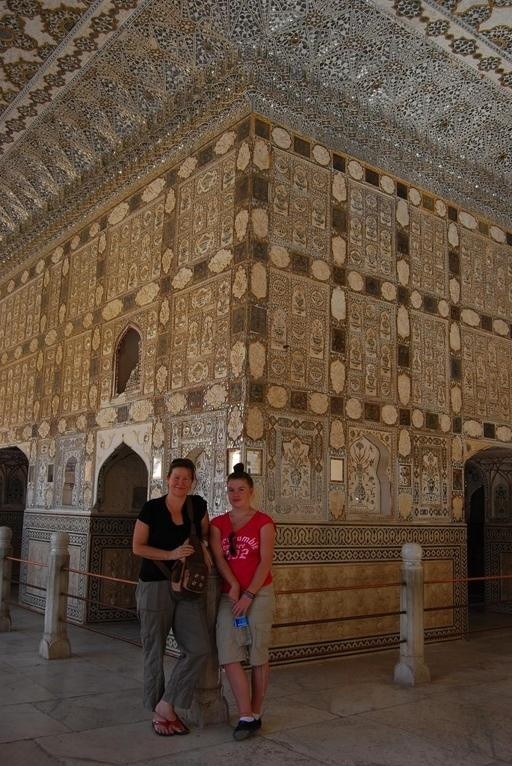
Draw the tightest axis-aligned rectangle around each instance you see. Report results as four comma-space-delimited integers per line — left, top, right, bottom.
132, 459, 213, 736
210, 463, 276, 742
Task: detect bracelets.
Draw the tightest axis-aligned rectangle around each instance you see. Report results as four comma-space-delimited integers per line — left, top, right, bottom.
243, 590, 256, 601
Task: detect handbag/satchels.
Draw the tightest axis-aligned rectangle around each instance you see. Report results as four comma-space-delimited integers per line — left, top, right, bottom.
168, 535, 209, 603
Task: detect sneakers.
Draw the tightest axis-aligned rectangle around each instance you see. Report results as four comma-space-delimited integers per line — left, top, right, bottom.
232, 718, 262, 740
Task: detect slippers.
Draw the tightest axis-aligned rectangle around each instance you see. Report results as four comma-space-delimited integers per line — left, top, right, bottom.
151, 710, 190, 738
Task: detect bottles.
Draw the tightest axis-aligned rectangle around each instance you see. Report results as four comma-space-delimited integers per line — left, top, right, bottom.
229, 600, 253, 648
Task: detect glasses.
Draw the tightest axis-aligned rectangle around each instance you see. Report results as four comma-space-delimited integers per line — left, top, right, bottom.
228, 532, 236, 557
